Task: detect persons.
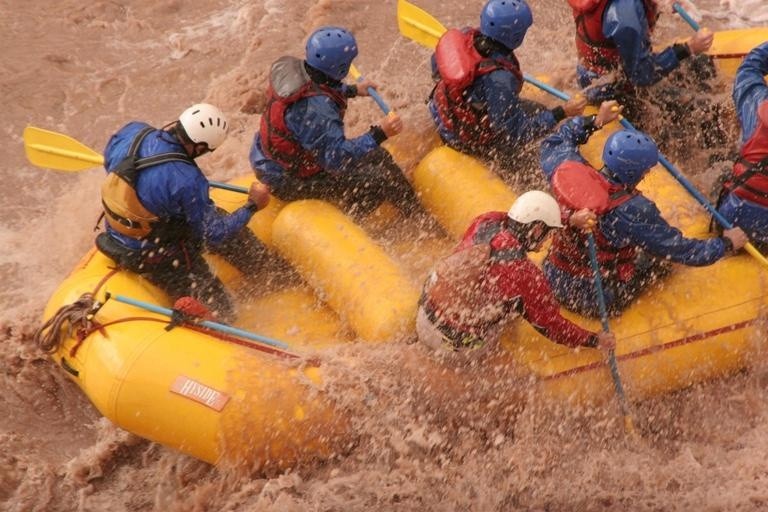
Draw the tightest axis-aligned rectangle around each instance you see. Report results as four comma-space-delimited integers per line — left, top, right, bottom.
426, 1, 586, 167
538, 98, 751, 322
414, 188, 617, 370
94, 100, 297, 324
247, 27, 444, 242
708, 41, 766, 255
569, 0, 731, 150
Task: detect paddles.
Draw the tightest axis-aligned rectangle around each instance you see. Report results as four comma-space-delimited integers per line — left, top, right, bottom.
583, 219, 644, 447
23, 126, 260, 195
348, 63, 396, 121
396, 1, 579, 106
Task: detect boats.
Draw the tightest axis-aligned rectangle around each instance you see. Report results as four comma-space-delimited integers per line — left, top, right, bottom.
41, 27, 767, 466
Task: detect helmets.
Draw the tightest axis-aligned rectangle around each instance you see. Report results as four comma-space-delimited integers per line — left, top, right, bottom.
602, 129, 658, 185
178, 104, 228, 152
304, 26, 358, 81
507, 190, 564, 229
480, 0, 533, 50
